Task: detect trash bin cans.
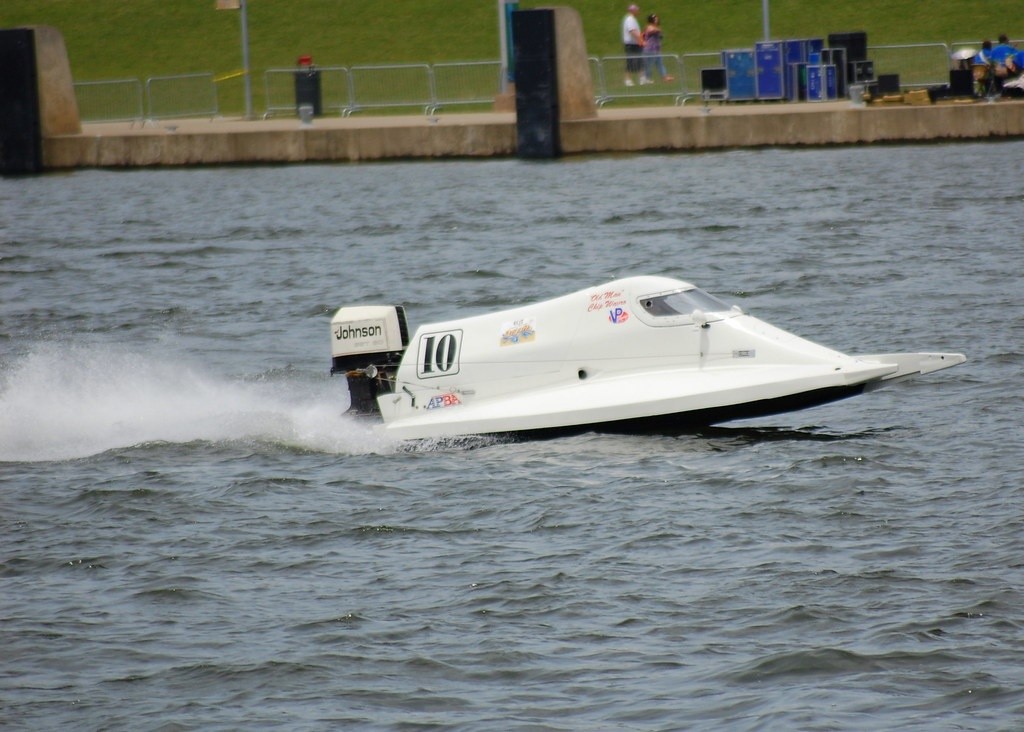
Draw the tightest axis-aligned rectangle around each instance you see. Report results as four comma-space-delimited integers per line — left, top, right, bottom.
293, 65, 322, 117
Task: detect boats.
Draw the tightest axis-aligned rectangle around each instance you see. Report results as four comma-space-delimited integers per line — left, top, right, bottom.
327, 274, 968, 442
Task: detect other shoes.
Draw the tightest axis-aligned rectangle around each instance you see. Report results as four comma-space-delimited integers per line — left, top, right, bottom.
625, 81, 635, 87
663, 75, 674, 82
640, 79, 654, 85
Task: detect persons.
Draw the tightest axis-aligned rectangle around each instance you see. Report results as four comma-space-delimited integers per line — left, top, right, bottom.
992, 34, 1019, 98
644, 14, 674, 81
971, 41, 992, 95
379, 350, 401, 373
1005, 50, 1024, 76
623, 5, 654, 86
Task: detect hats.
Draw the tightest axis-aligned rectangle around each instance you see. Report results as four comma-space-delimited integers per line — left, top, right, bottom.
647, 13, 656, 22
629, 4, 638, 11
